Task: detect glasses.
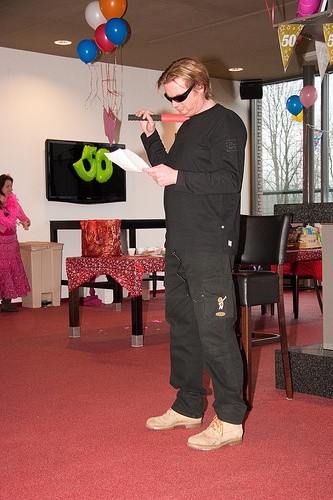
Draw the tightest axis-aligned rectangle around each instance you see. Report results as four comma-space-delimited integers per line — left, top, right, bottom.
164, 82, 196, 103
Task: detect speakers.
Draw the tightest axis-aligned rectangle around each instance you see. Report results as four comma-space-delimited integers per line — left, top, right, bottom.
240, 81, 263, 100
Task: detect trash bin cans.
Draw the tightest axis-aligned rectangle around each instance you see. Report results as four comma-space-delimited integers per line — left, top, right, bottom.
18, 241, 64, 308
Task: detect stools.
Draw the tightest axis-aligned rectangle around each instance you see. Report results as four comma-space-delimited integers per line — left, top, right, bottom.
232, 213, 292, 409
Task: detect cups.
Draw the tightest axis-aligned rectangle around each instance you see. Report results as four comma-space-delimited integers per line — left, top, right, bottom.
128, 248, 135, 255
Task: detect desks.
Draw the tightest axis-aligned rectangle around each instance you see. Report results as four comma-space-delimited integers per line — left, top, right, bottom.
66, 249, 322, 347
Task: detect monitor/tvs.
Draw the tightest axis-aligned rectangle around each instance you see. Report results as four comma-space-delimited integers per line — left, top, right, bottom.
45, 139, 126, 204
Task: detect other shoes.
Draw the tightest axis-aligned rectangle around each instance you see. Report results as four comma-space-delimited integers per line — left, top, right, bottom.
0, 302, 20, 313
186, 416, 244, 451
144, 407, 203, 431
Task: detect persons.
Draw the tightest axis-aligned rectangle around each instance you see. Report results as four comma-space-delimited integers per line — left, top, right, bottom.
136, 57, 248, 453
0, 175, 31, 312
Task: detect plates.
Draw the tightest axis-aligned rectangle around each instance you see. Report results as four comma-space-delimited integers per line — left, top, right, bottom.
129, 251, 166, 256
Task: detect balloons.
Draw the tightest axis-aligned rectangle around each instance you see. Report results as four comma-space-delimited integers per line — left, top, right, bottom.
78, 0, 128, 64
286, 85, 318, 122
296, 0, 321, 16
